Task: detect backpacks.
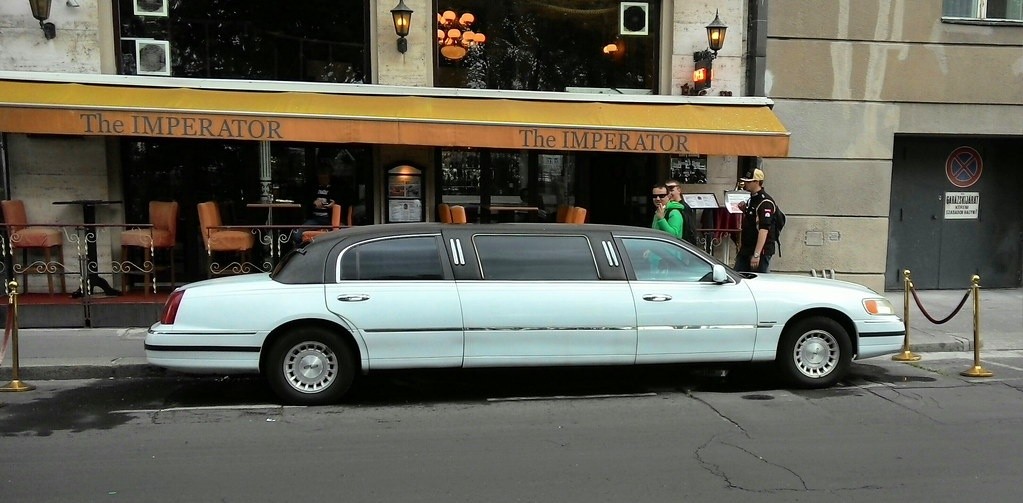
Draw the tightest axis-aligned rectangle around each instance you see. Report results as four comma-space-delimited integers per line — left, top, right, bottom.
775, 207, 786, 242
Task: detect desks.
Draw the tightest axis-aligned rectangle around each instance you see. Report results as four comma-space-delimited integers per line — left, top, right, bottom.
247, 203, 301, 225
480, 203, 539, 222
442, 195, 481, 203
491, 196, 522, 203
53, 200, 122, 298
541, 194, 575, 204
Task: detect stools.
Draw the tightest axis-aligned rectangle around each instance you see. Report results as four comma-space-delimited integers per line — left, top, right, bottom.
302, 204, 352, 244
121, 201, 178, 295
557, 204, 587, 224
438, 203, 466, 224
2, 199, 66, 297
196, 200, 255, 280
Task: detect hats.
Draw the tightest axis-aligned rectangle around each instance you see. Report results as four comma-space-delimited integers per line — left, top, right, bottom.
740, 168, 764, 181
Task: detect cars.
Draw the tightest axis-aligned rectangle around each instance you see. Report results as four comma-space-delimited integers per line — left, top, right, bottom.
143, 220, 907, 406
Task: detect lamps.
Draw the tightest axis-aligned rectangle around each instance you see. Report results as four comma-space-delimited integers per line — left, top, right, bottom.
704, 8, 728, 59
30, 0, 55, 40
390, 0, 414, 55
437, 11, 485, 60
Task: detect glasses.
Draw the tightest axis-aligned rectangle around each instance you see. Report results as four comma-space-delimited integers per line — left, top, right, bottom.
667, 186, 678, 190
652, 194, 668, 198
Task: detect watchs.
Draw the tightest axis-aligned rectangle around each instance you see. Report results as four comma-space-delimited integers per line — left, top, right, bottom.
753, 253, 759, 257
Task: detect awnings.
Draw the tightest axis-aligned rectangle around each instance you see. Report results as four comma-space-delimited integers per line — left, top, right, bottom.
0, 72, 791, 158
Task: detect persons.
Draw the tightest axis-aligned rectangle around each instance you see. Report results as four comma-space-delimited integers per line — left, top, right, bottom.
310, 169, 334, 221
735, 179, 745, 191
734, 168, 776, 273
643, 183, 683, 275
666, 180, 696, 246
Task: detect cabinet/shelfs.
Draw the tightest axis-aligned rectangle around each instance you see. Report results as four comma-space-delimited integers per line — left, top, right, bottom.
442, 150, 480, 188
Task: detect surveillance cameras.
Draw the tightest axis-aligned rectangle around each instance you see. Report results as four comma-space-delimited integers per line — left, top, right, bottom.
69, 0, 79, 7
